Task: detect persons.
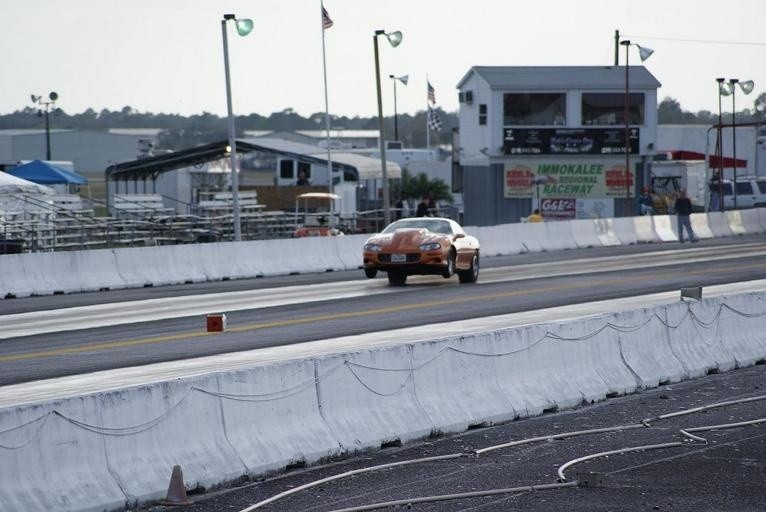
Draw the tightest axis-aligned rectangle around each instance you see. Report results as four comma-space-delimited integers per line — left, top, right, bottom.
708, 167, 722, 212
423, 192, 438, 217
415, 196, 430, 217
297, 170, 310, 187
526, 209, 544, 224
635, 186, 656, 215
673, 191, 700, 243
395, 190, 410, 219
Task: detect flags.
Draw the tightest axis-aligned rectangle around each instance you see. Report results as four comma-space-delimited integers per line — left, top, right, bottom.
428, 106, 442, 133
427, 82, 436, 104
321, 2, 333, 30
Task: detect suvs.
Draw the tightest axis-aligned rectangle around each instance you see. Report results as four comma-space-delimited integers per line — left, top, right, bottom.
708, 175, 766, 209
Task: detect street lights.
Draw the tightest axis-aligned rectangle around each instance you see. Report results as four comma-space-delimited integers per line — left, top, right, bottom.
373, 27, 406, 235
387, 71, 410, 145
220, 9, 256, 240
28, 88, 60, 159
716, 72, 754, 209
624, 34, 658, 219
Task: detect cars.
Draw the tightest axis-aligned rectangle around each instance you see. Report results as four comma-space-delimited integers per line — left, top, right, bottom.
140, 213, 225, 244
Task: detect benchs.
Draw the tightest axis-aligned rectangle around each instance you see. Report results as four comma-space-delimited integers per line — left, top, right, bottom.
0, 190, 305, 255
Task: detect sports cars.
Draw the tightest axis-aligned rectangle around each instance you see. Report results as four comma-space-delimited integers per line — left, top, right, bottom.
358, 217, 482, 285
